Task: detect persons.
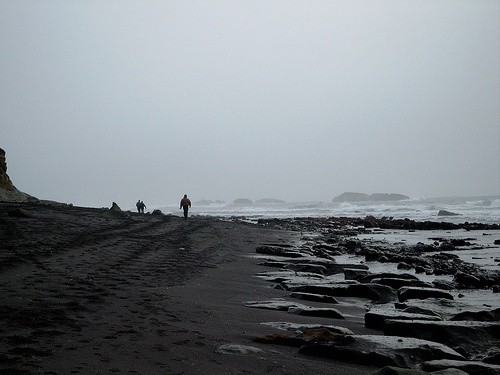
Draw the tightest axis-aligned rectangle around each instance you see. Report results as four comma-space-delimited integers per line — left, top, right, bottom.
140, 201, 146, 213
179, 194, 191, 217
136, 200, 141, 213
